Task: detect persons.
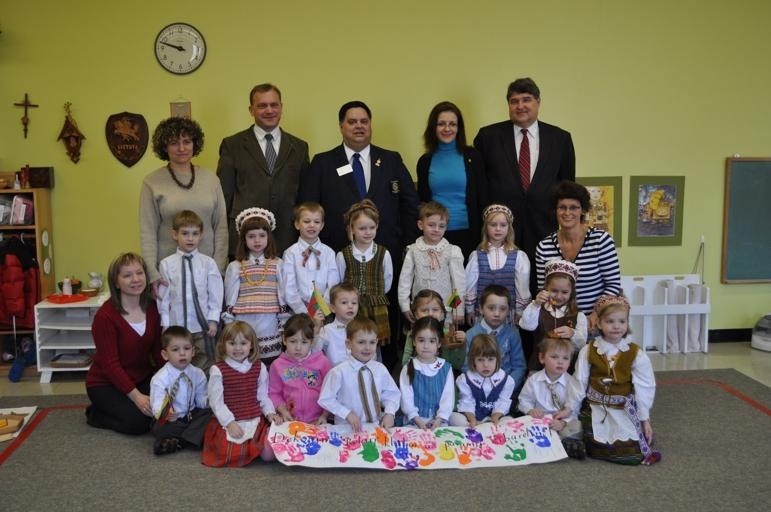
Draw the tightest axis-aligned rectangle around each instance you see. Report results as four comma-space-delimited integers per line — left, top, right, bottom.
139, 76, 578, 301
147, 261, 657, 466
83, 180, 622, 438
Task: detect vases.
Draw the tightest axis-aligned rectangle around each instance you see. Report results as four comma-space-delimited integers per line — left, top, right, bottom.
88, 271, 102, 290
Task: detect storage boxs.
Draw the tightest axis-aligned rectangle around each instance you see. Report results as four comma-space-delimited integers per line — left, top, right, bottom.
15, 167, 54, 188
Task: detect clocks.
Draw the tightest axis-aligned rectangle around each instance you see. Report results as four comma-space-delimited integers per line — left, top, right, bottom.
154, 22, 207, 75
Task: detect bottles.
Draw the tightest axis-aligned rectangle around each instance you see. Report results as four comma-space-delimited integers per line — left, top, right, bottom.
63, 278, 72, 298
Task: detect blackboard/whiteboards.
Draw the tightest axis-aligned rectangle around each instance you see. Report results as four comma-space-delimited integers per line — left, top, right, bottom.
721, 156, 771, 285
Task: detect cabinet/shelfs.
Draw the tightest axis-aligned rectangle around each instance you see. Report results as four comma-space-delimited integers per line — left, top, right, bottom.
34, 290, 111, 382
0, 187, 56, 378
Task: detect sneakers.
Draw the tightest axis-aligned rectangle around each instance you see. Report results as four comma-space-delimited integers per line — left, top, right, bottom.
153, 436, 189, 457
563, 438, 587, 461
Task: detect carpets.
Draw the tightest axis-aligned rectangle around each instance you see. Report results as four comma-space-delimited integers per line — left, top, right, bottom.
0, 368, 771, 512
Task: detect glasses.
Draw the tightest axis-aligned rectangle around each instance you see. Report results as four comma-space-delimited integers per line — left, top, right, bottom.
557, 204, 580, 210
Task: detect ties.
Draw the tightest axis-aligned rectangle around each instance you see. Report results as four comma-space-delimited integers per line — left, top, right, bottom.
264, 133, 278, 174
517, 128, 531, 190
547, 383, 562, 409
352, 152, 367, 202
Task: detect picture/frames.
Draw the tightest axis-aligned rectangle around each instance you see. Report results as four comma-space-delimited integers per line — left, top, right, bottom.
628, 176, 685, 246
577, 176, 622, 249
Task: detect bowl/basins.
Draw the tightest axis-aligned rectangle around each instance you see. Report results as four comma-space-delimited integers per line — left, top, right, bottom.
58, 281, 81, 294
